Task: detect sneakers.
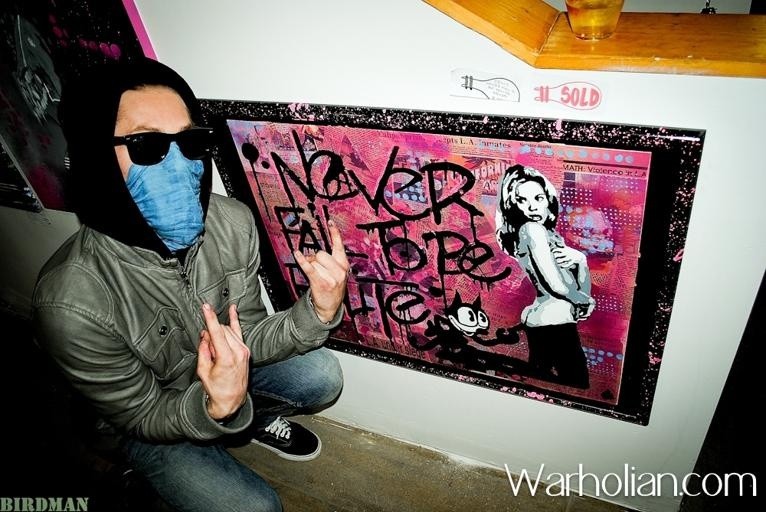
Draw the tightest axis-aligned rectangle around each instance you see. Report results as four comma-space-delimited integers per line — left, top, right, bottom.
250, 414, 323, 462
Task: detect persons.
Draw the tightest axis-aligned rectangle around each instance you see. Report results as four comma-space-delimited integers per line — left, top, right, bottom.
22, 50, 353, 512
435, 160, 597, 390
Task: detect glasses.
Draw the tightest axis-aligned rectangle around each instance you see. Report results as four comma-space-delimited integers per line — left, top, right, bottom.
111, 125, 219, 166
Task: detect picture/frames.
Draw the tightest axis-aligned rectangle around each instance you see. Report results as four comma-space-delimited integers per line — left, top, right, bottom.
191, 98, 707, 427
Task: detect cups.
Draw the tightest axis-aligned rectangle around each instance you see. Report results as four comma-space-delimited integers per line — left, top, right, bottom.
563, 0, 626, 42
